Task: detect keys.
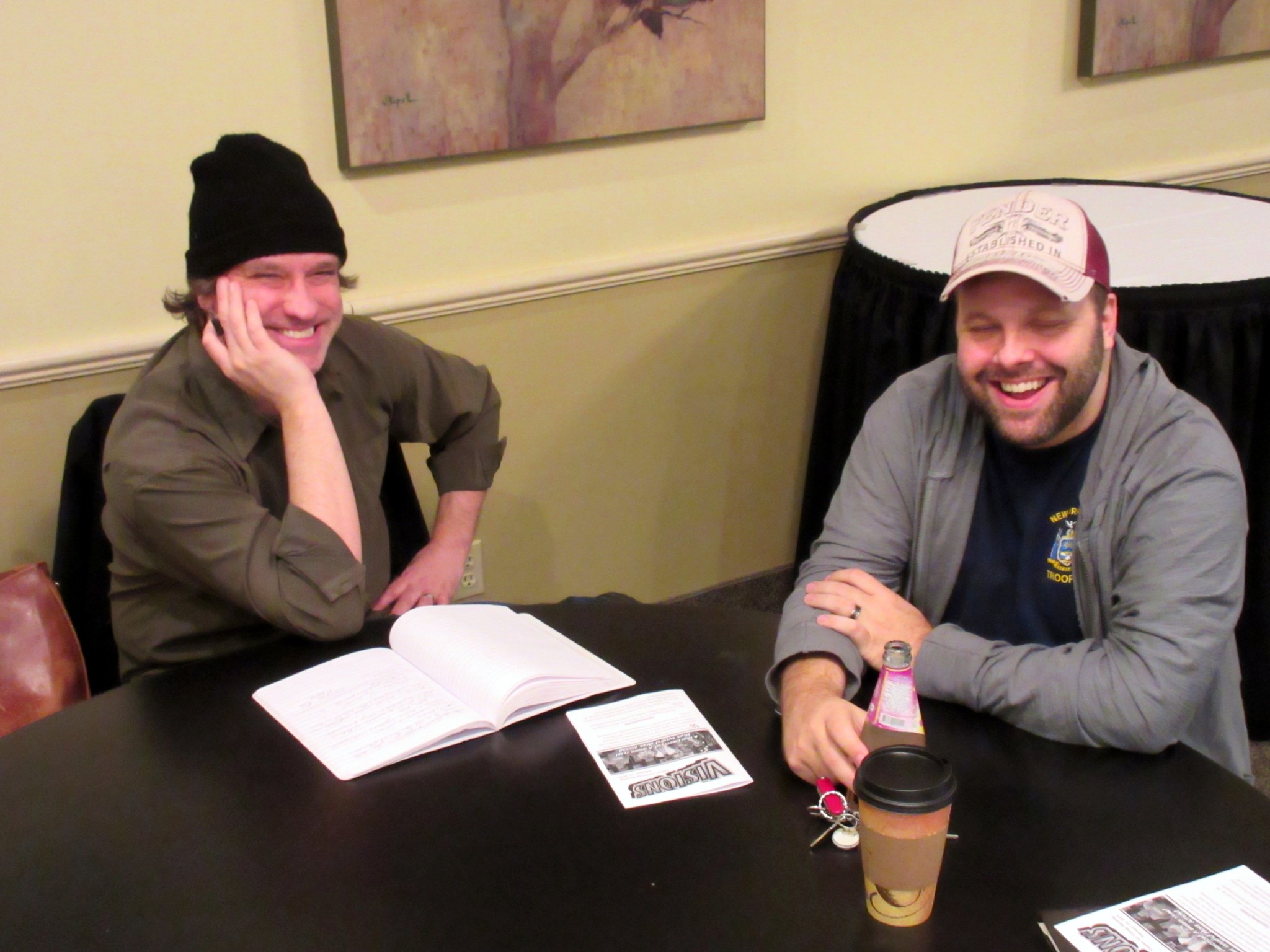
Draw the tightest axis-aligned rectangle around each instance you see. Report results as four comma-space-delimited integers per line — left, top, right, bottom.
806, 806, 845, 847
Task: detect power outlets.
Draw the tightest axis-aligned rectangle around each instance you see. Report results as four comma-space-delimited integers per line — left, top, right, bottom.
450, 541, 484, 604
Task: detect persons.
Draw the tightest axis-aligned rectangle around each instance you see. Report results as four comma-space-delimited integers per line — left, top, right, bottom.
761, 194, 1254, 799
99, 135, 507, 683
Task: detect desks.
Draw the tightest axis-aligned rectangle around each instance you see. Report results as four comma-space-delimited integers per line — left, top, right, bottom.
0, 555, 1270, 952
793, 178, 1270, 634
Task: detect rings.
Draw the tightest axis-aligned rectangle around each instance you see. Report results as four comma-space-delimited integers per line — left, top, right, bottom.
421, 594, 435, 601
849, 604, 861, 619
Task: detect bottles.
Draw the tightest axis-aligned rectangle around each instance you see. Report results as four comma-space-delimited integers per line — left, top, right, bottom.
845, 640, 925, 813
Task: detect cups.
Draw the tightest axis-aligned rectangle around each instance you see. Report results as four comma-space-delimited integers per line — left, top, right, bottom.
852, 744, 959, 927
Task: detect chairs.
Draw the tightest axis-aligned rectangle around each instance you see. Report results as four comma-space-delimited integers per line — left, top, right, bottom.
49, 392, 429, 698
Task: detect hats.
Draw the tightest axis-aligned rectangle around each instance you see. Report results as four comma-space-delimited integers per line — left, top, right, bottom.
940, 191, 1109, 302
184, 133, 347, 282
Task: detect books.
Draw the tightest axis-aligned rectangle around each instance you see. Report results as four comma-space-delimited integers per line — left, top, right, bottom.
252, 607, 635, 782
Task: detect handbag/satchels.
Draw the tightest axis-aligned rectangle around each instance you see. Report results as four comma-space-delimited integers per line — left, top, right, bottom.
0, 561, 90, 739
